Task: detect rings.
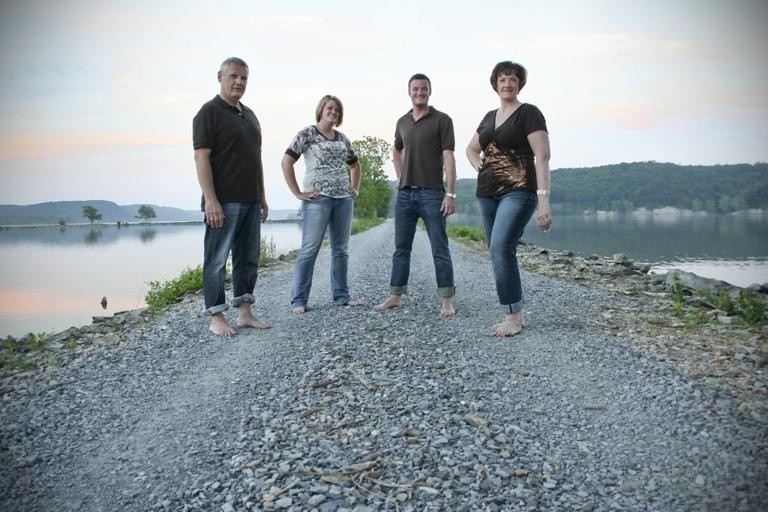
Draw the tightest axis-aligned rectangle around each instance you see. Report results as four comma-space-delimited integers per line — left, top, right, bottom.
209, 217, 214, 221
543, 216, 547, 219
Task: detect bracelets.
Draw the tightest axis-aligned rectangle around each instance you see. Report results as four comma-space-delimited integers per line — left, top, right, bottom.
445, 193, 457, 199
535, 189, 551, 197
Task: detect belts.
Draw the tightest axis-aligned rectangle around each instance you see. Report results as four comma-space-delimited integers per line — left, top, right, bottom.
400, 185, 435, 192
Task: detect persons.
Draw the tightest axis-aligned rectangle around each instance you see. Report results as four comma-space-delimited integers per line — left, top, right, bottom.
371, 72, 458, 318
280, 94, 364, 315
192, 57, 273, 337
464, 59, 554, 337
